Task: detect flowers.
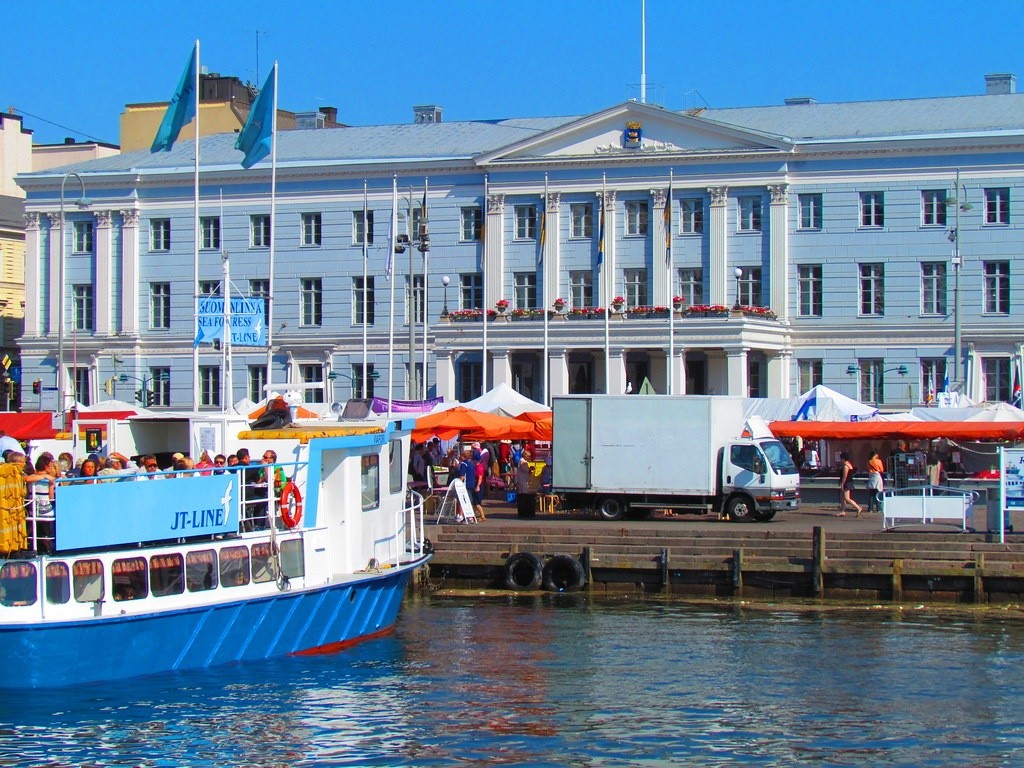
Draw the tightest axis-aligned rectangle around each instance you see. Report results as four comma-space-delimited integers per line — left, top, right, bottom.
447, 298, 776, 319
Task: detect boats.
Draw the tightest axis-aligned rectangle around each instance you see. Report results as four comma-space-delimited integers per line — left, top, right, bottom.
0, 417, 435, 690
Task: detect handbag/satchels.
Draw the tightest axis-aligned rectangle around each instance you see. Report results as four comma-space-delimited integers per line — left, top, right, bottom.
408, 453, 420, 475
940, 471, 948, 482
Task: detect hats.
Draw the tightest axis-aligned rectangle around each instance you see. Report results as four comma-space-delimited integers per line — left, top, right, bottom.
206, 450, 215, 464
107, 454, 120, 462
87, 453, 100, 465
173, 453, 184, 460
433, 438, 441, 443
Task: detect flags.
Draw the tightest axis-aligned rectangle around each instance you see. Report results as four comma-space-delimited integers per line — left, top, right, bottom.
385, 187, 397, 281
595, 191, 605, 272
234, 64, 274, 169
362, 190, 368, 263
943, 364, 951, 406
925, 371, 934, 408
659, 183, 671, 266
149, 44, 197, 152
537, 194, 545, 266
419, 191, 429, 273
479, 190, 486, 271
1012, 357, 1022, 410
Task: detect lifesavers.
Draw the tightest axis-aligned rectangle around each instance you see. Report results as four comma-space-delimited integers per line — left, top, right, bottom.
542, 555, 586, 592
503, 552, 544, 591
280, 482, 303, 528
251, 409, 292, 431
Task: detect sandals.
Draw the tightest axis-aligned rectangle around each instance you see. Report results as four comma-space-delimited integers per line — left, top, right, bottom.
477, 516, 487, 523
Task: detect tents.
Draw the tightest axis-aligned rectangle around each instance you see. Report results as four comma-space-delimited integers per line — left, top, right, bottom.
741, 384, 1024, 442
398, 383, 554, 440
219, 391, 379, 421
60, 399, 154, 422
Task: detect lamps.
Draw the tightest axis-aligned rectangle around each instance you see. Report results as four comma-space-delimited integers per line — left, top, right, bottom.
735, 268, 743, 278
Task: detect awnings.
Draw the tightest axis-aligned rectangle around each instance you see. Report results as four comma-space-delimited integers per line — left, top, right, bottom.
0, 413, 58, 438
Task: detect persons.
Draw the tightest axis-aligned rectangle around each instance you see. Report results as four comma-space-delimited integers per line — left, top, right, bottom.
891, 439, 947, 497
835, 452, 862, 517
782, 437, 821, 483
0, 430, 285, 550
410, 440, 553, 523
865, 449, 884, 512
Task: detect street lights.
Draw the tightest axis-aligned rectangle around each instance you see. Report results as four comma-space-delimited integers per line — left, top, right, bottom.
942, 165, 973, 382
389, 196, 430, 402
326, 370, 381, 398
845, 364, 910, 408
57, 171, 93, 413
119, 372, 171, 408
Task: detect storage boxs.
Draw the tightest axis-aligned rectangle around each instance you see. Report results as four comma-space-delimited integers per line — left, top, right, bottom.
506, 491, 517, 503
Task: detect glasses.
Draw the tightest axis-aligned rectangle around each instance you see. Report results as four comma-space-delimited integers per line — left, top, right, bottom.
148, 464, 157, 467
263, 456, 272, 459
215, 461, 224, 465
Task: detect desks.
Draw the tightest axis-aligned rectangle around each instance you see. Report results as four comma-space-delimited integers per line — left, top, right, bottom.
540, 494, 561, 514
407, 480, 428, 505
432, 486, 450, 517
500, 472, 517, 495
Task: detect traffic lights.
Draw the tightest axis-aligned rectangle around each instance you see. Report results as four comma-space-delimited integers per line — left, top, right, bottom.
10, 380, 17, 400
33, 382, 40, 394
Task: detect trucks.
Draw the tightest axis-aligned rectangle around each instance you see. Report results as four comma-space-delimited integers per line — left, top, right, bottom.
549, 394, 801, 524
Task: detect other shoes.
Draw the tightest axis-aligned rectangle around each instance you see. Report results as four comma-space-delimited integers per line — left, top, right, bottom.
455, 514, 463, 522
835, 512, 847, 517
869, 509, 882, 513
855, 507, 862, 518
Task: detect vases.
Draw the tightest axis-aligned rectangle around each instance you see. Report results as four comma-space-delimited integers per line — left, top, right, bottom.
511, 316, 532, 321
626, 313, 648, 319
567, 314, 586, 320
673, 302, 681, 309
532, 315, 552, 321
650, 313, 670, 318
687, 312, 706, 317
495, 306, 506, 313
455, 317, 475, 322
612, 303, 622, 311
553, 305, 563, 311
476, 315, 496, 321
708, 313, 729, 317
591, 314, 611, 319
